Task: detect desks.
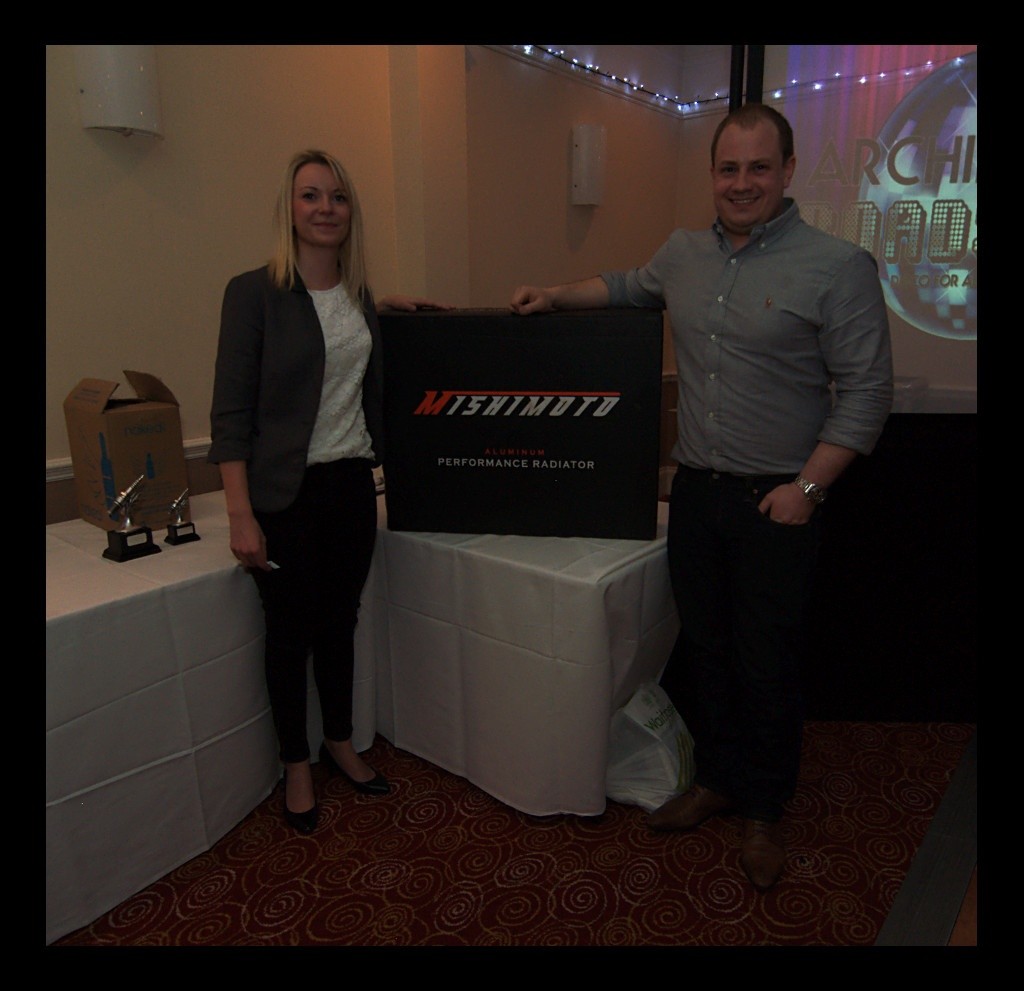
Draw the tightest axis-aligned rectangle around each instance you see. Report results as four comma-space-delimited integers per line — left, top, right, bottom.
44, 463, 385, 949
371, 478, 684, 821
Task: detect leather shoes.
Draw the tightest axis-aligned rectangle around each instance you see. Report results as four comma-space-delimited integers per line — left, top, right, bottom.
741, 817, 784, 889
646, 786, 729, 832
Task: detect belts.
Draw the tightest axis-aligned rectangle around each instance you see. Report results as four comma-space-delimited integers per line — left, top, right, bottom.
675, 464, 799, 486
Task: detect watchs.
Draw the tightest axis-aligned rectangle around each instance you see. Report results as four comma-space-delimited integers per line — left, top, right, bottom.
793, 474, 828, 505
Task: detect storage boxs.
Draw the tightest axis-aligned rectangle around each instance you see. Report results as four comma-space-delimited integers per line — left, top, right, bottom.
61, 367, 191, 530
376, 289, 664, 540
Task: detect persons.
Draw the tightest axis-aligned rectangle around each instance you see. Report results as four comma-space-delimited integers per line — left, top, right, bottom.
504, 106, 896, 893
206, 150, 454, 834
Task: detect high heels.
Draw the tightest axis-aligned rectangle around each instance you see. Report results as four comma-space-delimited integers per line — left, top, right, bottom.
282, 766, 317, 834
319, 742, 390, 795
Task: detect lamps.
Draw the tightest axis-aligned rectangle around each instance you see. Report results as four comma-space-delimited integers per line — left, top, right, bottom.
78, 44, 163, 142
572, 120, 605, 207
